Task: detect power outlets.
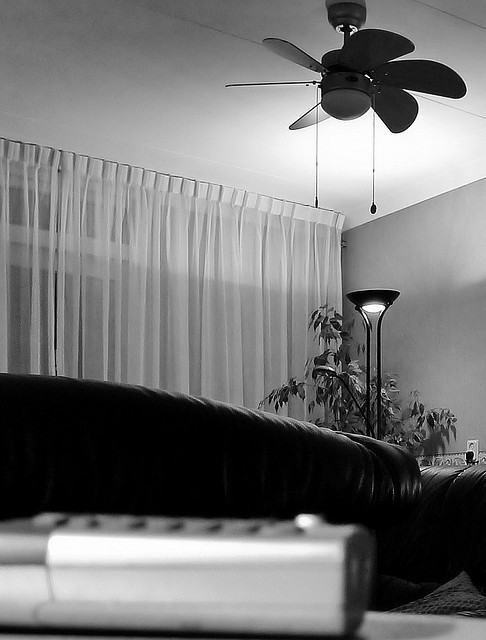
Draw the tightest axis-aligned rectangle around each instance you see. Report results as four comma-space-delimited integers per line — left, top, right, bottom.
465, 439, 479, 463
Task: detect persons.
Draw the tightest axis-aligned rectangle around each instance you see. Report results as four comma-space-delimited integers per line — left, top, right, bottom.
467, 442, 475, 461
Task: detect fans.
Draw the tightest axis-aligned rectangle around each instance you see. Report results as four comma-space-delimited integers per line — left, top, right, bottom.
220, 0, 467, 132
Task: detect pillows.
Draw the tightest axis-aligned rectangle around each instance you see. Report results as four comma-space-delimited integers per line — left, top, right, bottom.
378, 571, 485, 622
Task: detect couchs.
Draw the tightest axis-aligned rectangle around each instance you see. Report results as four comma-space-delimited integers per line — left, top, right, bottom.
0, 373, 485, 635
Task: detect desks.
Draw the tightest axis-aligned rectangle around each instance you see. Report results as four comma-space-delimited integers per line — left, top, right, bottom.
358, 609, 486, 640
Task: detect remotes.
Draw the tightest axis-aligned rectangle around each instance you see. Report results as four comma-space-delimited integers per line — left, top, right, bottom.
0, 510, 373, 634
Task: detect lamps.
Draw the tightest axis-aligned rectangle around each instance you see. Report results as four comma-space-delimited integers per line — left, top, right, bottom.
346, 282, 400, 445
